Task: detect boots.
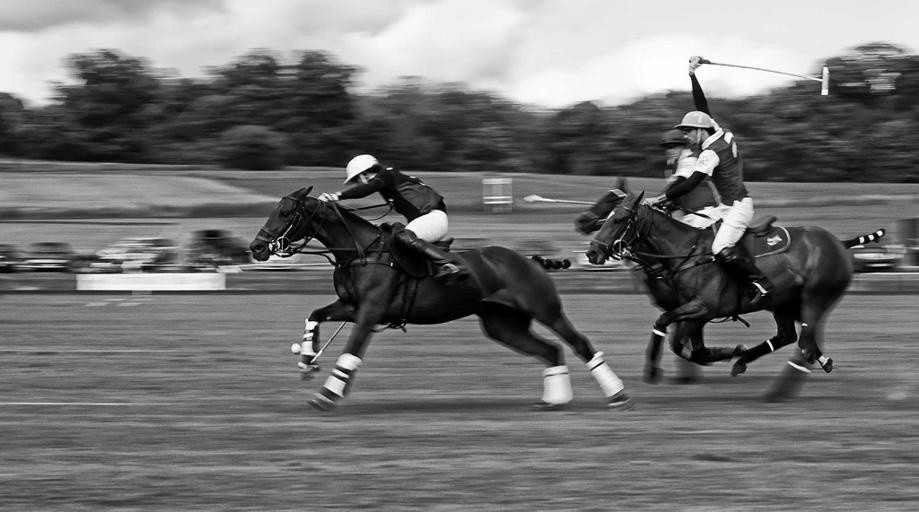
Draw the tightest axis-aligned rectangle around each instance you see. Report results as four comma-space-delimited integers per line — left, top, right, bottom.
715, 247, 777, 305
396, 227, 467, 282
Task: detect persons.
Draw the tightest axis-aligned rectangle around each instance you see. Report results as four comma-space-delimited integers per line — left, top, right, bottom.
642, 55, 776, 309
319, 152, 469, 284
656, 128, 723, 234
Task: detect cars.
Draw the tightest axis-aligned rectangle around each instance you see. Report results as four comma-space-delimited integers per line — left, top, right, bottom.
0, 229, 233, 275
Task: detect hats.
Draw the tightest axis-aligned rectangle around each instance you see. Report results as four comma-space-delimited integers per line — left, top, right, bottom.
659, 128, 691, 148
674, 109, 717, 131
342, 153, 380, 186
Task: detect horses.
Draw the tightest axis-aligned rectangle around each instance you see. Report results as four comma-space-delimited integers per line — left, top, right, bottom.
249, 185, 633, 413
575, 177, 857, 403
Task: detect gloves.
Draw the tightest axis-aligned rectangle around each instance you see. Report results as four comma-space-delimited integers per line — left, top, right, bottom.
687, 54, 706, 73
317, 191, 344, 205
642, 196, 659, 206
